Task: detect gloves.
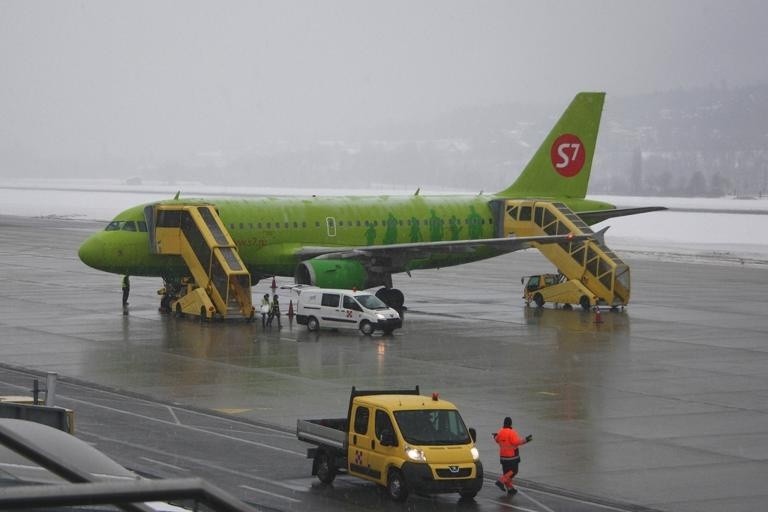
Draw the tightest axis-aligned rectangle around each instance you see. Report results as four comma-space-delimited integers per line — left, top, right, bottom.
526, 435, 532, 443
492, 433, 497, 438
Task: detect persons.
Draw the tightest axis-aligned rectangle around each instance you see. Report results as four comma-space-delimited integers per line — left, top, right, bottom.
270, 294, 283, 328
259, 293, 272, 330
120, 274, 130, 304
491, 415, 533, 495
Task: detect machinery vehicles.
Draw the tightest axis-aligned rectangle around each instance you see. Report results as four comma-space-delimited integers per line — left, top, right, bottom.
520, 273, 607, 313
156, 282, 221, 323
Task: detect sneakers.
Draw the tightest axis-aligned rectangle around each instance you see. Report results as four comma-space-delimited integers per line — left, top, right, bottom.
495, 480, 517, 494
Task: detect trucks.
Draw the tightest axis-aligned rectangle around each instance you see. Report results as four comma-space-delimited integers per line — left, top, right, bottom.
295, 384, 484, 502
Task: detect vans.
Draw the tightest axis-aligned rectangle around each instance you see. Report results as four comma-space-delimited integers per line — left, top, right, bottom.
295, 286, 403, 336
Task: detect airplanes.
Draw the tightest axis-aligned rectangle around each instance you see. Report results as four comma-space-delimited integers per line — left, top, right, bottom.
77, 91, 670, 311
